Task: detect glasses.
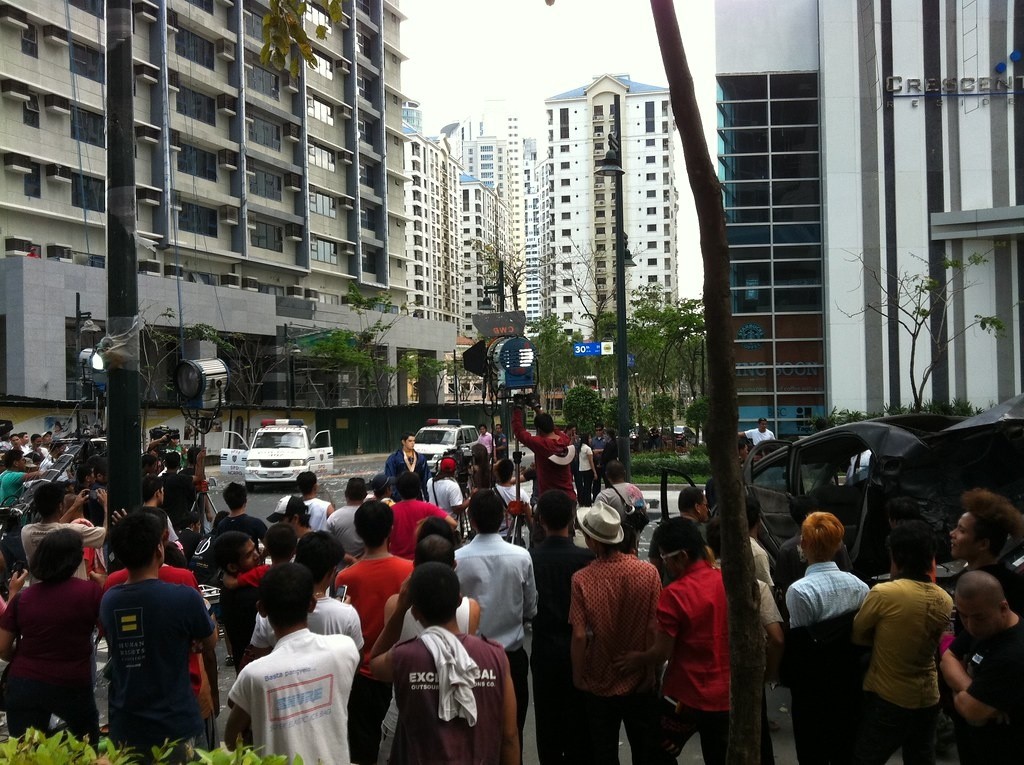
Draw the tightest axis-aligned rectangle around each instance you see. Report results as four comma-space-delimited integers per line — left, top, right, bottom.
595, 428, 603, 431
660, 551, 687, 564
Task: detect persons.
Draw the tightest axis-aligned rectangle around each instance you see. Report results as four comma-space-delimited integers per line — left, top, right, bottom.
0, 433, 364, 711
784, 512, 872, 765
939, 570, 1024, 765
224, 560, 364, 765
99, 514, 218, 765
852, 496, 952, 765
564, 420, 619, 509
531, 489, 598, 765
708, 495, 784, 765
369, 563, 522, 765
386, 433, 430, 502
0, 529, 105, 765
650, 486, 715, 586
595, 458, 649, 559
738, 418, 781, 467
494, 422, 510, 459
471, 424, 494, 462
454, 489, 538, 765
936, 486, 1024, 660
567, 501, 663, 765
53, 421, 63, 438
511, 398, 578, 541
327, 458, 537, 765
612, 516, 732, 765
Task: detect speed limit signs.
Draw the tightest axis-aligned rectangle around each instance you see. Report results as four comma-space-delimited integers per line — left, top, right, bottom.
573, 343, 601, 357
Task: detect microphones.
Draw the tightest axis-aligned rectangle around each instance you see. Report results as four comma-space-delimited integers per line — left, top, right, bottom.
170, 429, 179, 433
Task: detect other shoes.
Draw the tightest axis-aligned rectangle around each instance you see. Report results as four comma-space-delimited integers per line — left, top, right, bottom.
226, 655, 234, 667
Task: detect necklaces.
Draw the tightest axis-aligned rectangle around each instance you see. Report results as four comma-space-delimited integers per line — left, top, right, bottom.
313, 591, 324, 598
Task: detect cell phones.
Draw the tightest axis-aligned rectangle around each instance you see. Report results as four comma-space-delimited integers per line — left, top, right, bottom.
336, 585, 347, 602
85, 490, 98, 500
13, 560, 25, 578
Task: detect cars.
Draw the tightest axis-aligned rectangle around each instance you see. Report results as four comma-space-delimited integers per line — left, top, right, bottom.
662, 391, 1024, 582
615, 425, 697, 458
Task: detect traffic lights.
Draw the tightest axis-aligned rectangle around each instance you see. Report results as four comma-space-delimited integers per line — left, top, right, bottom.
172, 357, 230, 434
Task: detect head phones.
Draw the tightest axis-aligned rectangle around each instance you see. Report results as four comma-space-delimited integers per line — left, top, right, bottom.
435, 456, 460, 476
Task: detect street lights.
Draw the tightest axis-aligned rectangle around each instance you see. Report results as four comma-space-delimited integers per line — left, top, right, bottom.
479, 260, 506, 314
594, 93, 631, 482
75, 292, 102, 437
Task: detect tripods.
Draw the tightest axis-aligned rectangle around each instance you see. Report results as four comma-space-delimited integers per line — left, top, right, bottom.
191, 481, 218, 535
506, 437, 532, 545
455, 483, 470, 539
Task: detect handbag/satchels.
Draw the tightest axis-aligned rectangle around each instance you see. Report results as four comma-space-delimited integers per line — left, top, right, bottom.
611, 484, 649, 532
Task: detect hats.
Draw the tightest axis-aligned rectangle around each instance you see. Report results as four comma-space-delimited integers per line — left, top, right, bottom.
441, 459, 456, 474
577, 501, 625, 544
266, 495, 305, 523
545, 445, 576, 466
371, 474, 397, 492
41, 431, 52, 440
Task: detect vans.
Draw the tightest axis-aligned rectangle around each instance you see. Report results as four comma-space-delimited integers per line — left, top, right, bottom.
413, 419, 480, 478
221, 419, 334, 494
